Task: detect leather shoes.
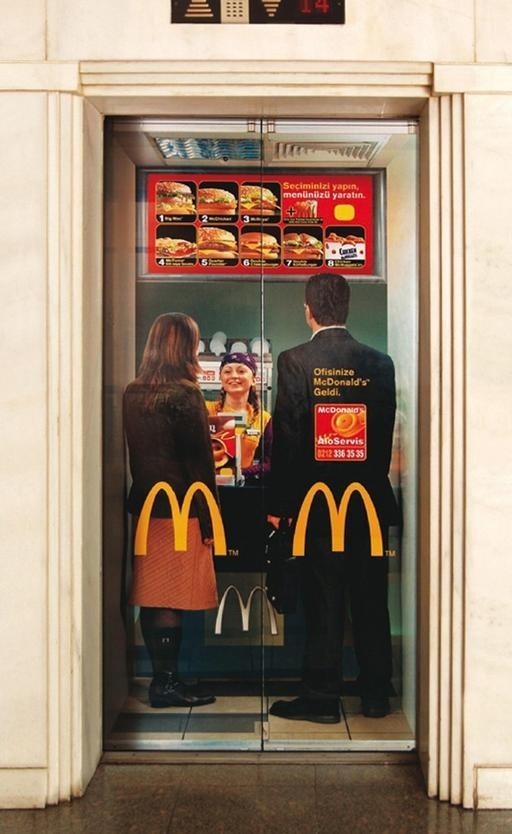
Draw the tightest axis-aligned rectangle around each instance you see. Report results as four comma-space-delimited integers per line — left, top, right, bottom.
270, 697, 341, 724
362, 697, 390, 717
150, 673, 216, 707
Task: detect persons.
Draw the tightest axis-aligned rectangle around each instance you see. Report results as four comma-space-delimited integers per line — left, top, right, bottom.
203, 349, 275, 478
260, 270, 402, 725
120, 309, 224, 707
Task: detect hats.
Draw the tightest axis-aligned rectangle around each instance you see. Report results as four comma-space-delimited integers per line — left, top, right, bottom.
220, 352, 257, 375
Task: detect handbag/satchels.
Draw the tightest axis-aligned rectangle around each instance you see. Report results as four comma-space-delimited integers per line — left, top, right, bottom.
266, 540, 299, 614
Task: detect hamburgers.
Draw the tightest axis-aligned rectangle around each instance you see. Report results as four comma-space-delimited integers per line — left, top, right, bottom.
155, 181, 323, 259
